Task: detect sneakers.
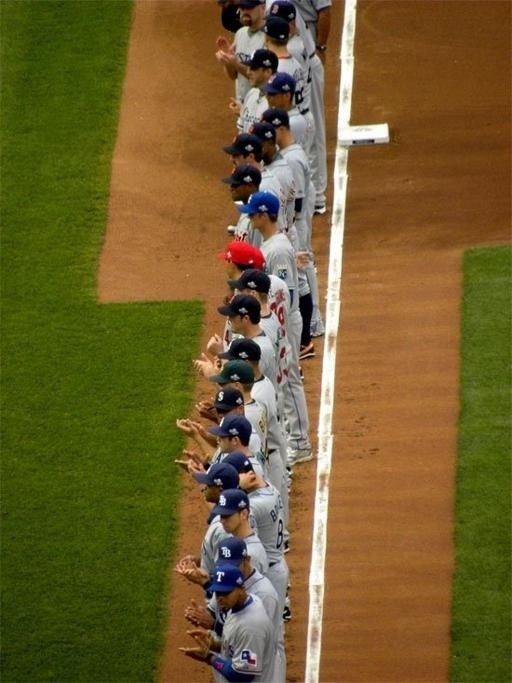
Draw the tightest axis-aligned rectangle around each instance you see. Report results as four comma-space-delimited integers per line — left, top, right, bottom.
298, 342, 314, 359
285, 446, 313, 467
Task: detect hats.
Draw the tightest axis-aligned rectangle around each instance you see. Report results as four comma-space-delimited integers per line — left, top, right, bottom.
192, 240, 270, 593
221, 1, 297, 213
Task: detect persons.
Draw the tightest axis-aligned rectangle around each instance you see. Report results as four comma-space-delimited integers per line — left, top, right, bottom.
216, 1, 334, 464
171, 240, 345, 682
